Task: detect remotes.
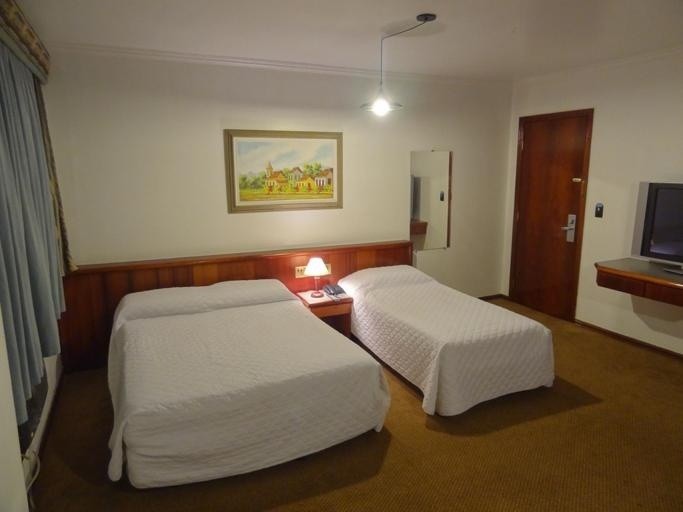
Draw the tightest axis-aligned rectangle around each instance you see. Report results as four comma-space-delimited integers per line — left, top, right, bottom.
328, 294, 340, 302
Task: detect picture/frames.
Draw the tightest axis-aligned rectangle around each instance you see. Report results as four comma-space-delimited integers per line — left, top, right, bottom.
223, 128, 346, 214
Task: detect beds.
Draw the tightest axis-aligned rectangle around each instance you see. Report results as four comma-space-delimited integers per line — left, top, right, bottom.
117, 268, 390, 488
336, 268, 556, 417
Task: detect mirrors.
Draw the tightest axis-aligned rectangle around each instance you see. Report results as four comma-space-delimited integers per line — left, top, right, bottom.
409, 148, 452, 251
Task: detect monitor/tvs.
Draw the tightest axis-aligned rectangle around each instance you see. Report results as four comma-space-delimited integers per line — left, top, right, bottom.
410, 174, 415, 220
630, 180, 683, 276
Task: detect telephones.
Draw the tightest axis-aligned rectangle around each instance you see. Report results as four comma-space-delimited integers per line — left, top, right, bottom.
324, 284, 344, 295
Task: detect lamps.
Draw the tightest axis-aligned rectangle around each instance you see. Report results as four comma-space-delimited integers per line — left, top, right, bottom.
360, 11, 437, 118
303, 255, 330, 298
